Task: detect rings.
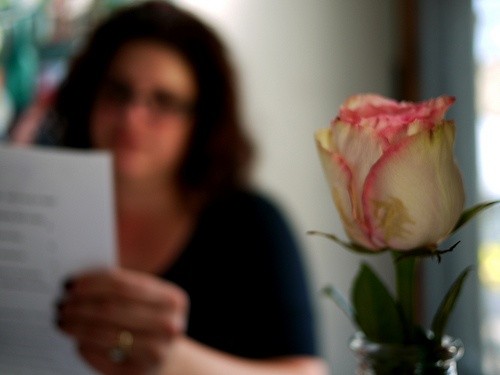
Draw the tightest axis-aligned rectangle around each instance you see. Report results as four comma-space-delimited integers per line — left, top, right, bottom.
109, 331, 135, 362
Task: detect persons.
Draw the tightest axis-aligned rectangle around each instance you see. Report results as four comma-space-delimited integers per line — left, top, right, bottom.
26, 1, 327, 374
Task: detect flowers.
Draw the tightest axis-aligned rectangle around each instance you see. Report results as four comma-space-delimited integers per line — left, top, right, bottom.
301, 93, 500, 375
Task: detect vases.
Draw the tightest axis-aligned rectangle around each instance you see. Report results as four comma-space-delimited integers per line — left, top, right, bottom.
349, 325, 466, 375
